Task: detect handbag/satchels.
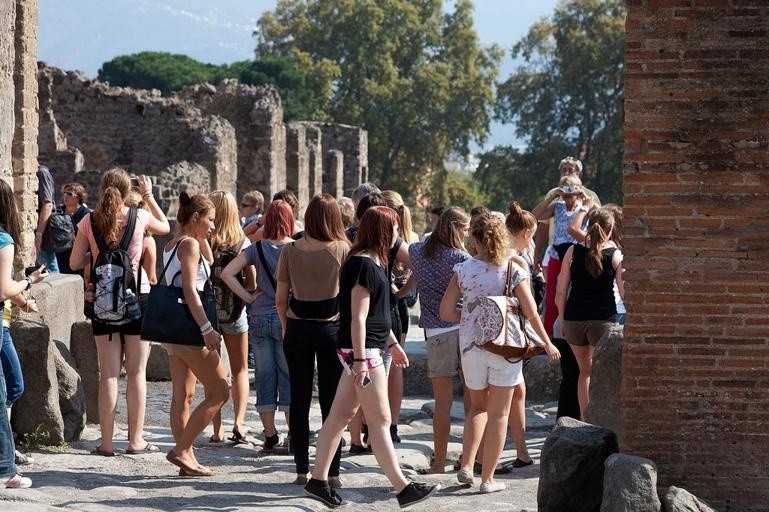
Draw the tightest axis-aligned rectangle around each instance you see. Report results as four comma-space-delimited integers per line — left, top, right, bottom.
141, 284, 218, 346
474, 293, 547, 364
43, 213, 75, 252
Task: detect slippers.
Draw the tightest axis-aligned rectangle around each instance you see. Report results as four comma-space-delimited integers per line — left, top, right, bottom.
125, 441, 159, 453
90, 445, 115, 456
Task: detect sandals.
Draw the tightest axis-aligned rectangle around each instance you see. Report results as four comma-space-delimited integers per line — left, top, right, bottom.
417, 456, 534, 493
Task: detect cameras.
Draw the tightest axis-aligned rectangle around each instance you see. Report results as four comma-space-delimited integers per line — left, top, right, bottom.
564, 186, 575, 193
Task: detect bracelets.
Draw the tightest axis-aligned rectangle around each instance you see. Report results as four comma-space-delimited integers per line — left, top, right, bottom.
352, 357, 367, 363
19, 302, 29, 308
25, 275, 33, 292
387, 343, 400, 348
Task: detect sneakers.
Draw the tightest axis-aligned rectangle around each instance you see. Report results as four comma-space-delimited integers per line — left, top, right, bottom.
210, 424, 400, 507
15, 451, 33, 465
0, 474, 31, 490
167, 449, 212, 477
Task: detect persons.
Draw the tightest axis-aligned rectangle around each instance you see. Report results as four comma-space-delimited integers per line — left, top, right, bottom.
67, 155, 627, 493
36, 160, 59, 273
303, 205, 442, 507
1, 228, 40, 470
0, 177, 52, 488
55, 180, 93, 281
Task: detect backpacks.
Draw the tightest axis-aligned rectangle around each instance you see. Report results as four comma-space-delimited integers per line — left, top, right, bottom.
83, 211, 142, 325
204, 239, 247, 328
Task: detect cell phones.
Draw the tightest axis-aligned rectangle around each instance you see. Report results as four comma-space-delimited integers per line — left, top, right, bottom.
130, 178, 139, 187
362, 376, 372, 388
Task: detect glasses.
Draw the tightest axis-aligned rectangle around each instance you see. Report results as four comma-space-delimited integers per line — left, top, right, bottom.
63, 191, 78, 198
241, 203, 253, 207
